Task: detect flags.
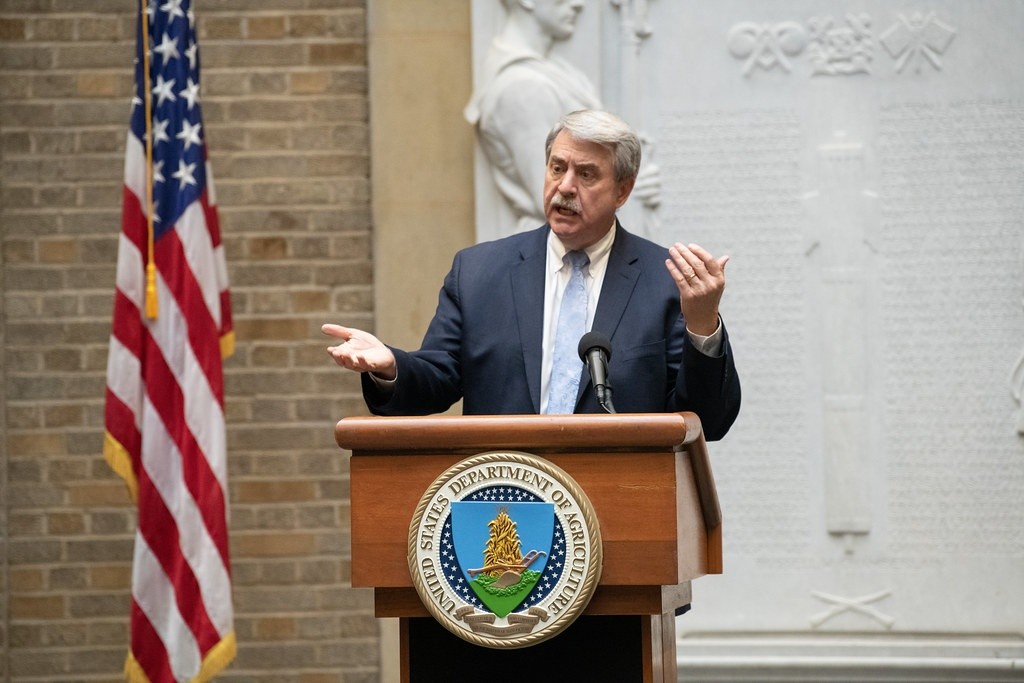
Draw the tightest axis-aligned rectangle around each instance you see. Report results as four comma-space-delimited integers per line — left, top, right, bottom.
99, 0, 240, 683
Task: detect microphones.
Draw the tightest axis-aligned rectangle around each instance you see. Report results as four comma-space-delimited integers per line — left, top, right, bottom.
578, 331, 613, 404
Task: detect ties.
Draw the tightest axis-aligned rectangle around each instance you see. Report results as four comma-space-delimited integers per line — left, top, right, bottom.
545, 250, 592, 415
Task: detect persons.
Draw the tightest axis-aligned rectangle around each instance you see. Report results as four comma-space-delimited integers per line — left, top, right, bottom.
317, 108, 741, 618
459, 0, 662, 241
